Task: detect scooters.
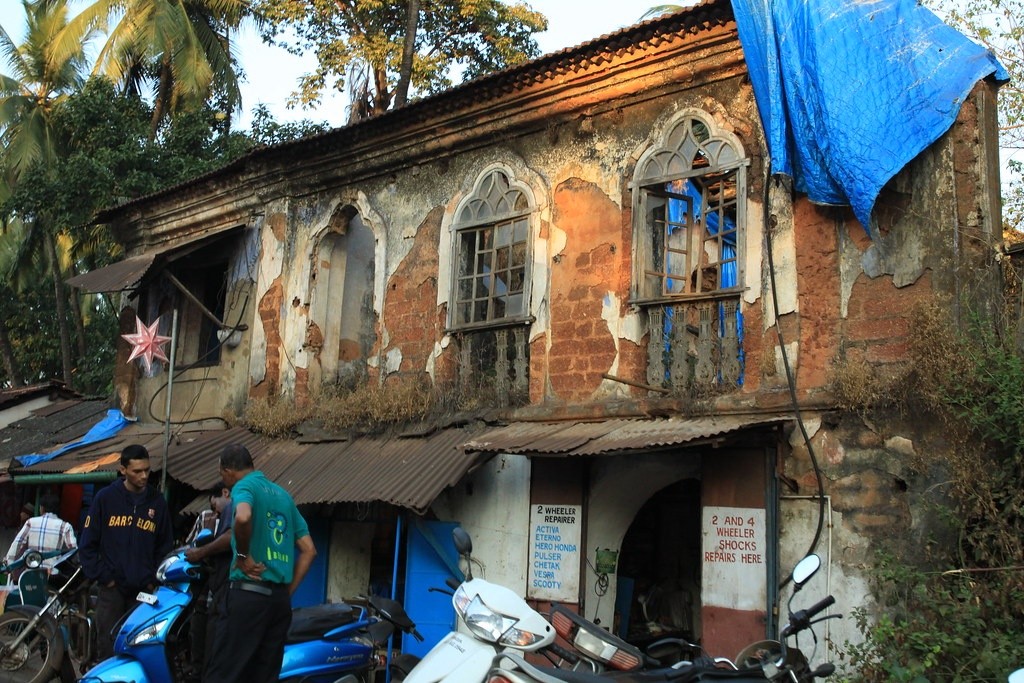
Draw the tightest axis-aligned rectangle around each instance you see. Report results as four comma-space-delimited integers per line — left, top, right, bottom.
396, 527, 844, 683
0, 535, 114, 683
79, 527, 416, 683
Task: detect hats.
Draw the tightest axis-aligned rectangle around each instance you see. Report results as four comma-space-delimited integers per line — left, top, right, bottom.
18, 502, 35, 516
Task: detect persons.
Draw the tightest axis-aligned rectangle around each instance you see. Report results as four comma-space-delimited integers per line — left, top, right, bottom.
77, 445, 171, 663
3, 494, 78, 586
184, 443, 316, 683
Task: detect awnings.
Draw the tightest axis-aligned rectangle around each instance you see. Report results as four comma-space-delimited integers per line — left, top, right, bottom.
12, 412, 802, 520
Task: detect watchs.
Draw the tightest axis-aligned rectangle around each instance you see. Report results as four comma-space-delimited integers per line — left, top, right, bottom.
237, 552, 250, 560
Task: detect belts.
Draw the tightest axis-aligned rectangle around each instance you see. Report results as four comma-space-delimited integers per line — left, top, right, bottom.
229, 580, 289, 595
